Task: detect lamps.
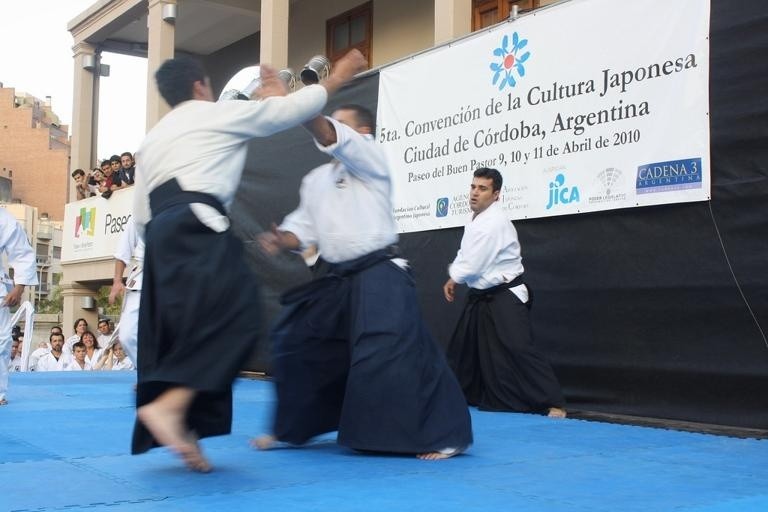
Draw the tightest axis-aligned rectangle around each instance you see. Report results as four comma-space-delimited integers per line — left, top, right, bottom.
299, 55, 331, 86
163, 3, 177, 23
82, 295, 95, 310
279, 68, 297, 88
237, 77, 263, 100
83, 54, 97, 71
217, 88, 240, 103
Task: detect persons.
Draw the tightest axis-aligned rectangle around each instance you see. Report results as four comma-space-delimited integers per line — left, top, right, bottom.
71, 152, 136, 199
109, 224, 151, 368
131, 46, 367, 473
443, 167, 567, 419
249, 64, 472, 460
0, 205, 37, 406
11, 318, 135, 373
